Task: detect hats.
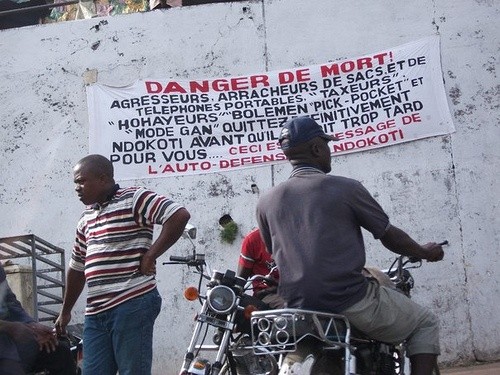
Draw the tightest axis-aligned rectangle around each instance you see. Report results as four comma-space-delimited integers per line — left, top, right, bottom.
279, 116, 338, 150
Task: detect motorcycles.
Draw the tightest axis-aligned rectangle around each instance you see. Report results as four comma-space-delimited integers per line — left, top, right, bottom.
162, 222, 278, 375
249, 240, 450, 375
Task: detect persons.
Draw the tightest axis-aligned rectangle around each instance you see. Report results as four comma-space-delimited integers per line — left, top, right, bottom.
235, 228, 284, 309
55, 154, 191, 375
255, 117, 444, 375
0, 261, 59, 375
